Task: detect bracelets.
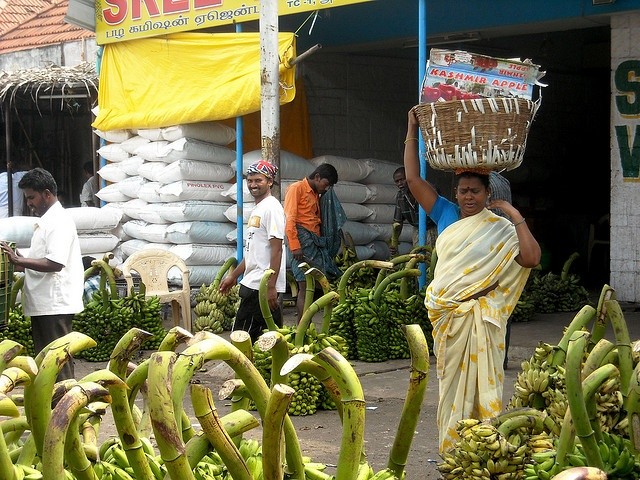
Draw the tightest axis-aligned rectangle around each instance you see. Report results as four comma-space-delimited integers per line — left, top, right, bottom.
514, 218, 527, 227
405, 138, 419, 144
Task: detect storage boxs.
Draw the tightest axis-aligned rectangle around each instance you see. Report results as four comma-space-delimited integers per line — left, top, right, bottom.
0, 241, 16, 287
1, 283, 11, 327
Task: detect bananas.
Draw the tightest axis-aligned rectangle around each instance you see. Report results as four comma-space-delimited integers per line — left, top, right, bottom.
8, 434, 166, 480
437, 324, 640, 480
327, 249, 435, 363
249, 321, 350, 417
507, 271, 593, 326
0, 304, 37, 355
356, 461, 396, 480
283, 454, 334, 480
72, 285, 168, 363
190, 438, 264, 480
192, 282, 241, 335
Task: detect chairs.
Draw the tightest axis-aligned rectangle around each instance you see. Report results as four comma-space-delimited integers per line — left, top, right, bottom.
121, 247, 193, 337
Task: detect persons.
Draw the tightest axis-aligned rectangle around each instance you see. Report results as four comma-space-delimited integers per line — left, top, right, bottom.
391, 166, 448, 246
404, 105, 541, 460
0, 168, 84, 409
284, 163, 338, 335
79, 161, 99, 207
0, 171, 35, 218
219, 160, 286, 397
